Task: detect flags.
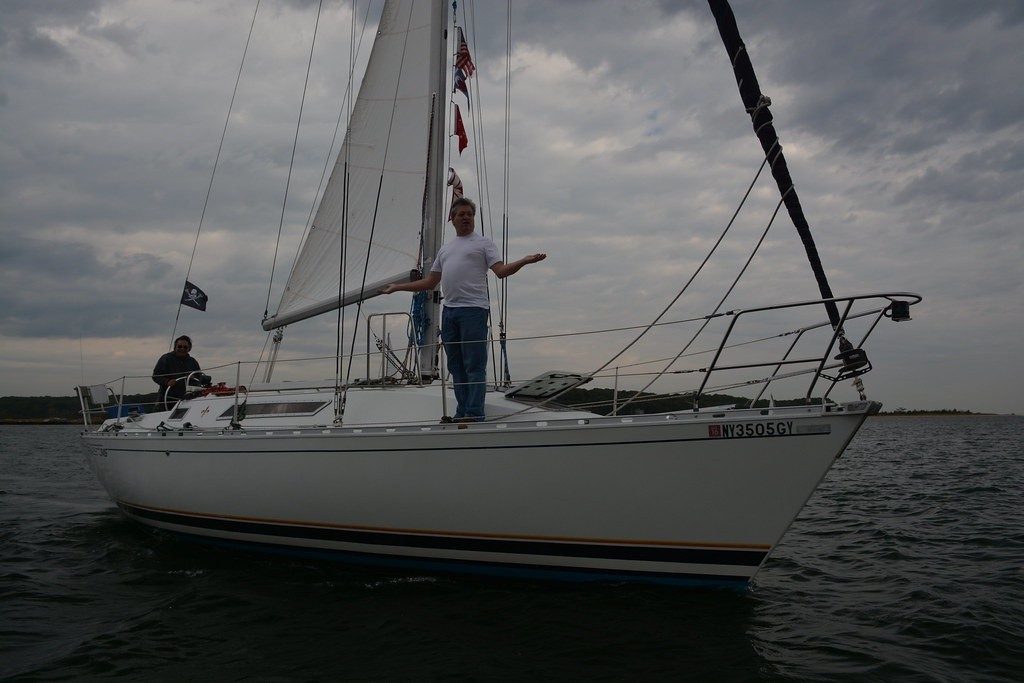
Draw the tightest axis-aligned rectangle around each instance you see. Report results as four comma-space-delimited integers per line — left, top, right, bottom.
454, 67, 470, 110
453, 105, 468, 156
448, 167, 464, 223
181, 281, 208, 312
456, 27, 475, 76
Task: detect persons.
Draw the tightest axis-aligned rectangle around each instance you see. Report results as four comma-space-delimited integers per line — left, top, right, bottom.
378, 197, 548, 420
151, 334, 202, 413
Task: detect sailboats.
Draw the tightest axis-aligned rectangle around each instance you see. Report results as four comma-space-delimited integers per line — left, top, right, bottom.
72, 0, 922, 594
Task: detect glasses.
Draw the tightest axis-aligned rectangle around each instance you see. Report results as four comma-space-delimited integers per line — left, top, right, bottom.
177, 345, 190, 349
